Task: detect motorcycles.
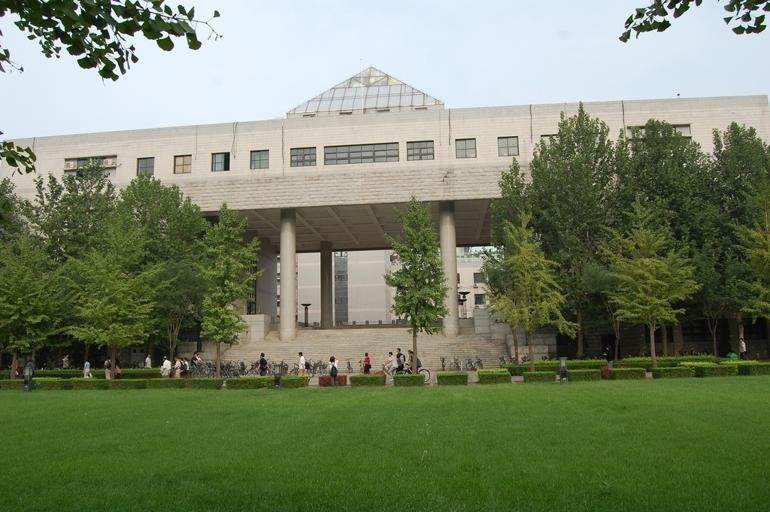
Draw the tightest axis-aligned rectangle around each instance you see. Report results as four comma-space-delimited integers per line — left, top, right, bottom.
599, 344, 612, 360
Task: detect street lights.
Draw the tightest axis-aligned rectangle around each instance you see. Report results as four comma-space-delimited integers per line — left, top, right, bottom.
301, 303, 311, 326
458, 290, 471, 319
458, 298, 467, 318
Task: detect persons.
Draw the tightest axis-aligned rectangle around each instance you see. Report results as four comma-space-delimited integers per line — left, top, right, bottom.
104, 356, 111, 379
382, 349, 422, 374
145, 353, 151, 368
298, 352, 305, 376
260, 353, 267, 376
9, 355, 35, 378
363, 353, 371, 374
115, 358, 121, 379
739, 338, 746, 360
84, 359, 90, 378
159, 352, 202, 379
63, 353, 72, 369
328, 356, 338, 386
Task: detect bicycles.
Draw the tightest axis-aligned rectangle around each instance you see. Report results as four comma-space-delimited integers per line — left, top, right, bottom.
357, 358, 364, 373
435, 354, 528, 372
345, 357, 353, 373
395, 364, 431, 383
189, 359, 329, 379
